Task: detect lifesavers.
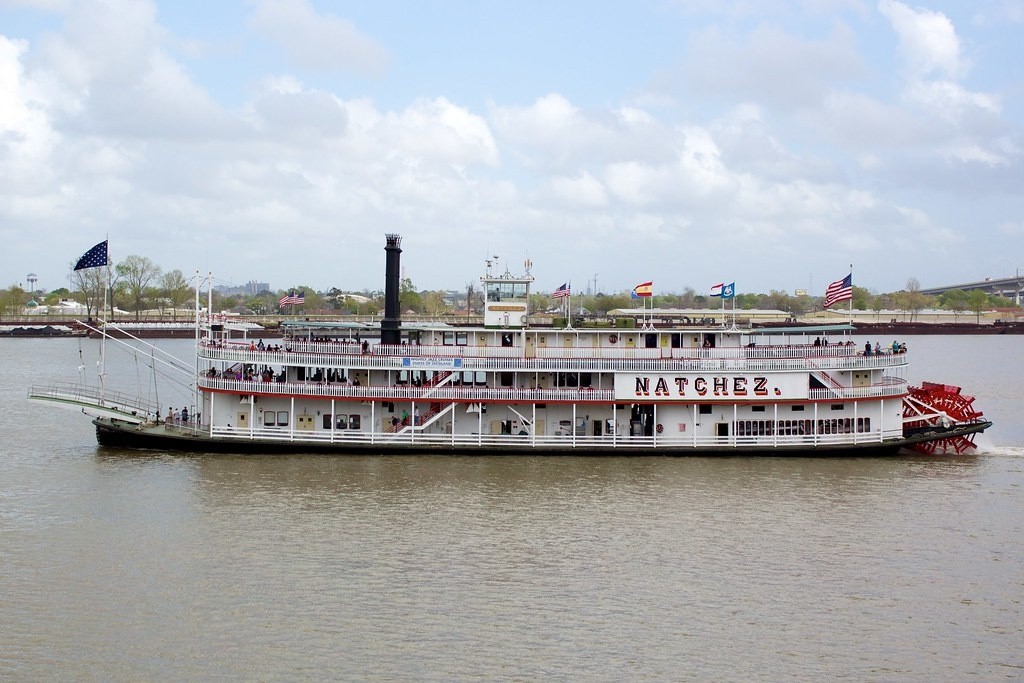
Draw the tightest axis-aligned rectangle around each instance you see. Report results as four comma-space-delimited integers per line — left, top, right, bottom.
609, 334, 618, 343
520, 315, 527, 323
657, 424, 663, 432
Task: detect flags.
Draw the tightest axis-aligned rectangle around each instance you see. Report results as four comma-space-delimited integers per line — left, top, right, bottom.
74, 239, 108, 271
710, 284, 723, 297
721, 282, 736, 298
630, 281, 653, 299
824, 274, 852, 307
279, 290, 295, 308
290, 292, 304, 304
552, 282, 570, 299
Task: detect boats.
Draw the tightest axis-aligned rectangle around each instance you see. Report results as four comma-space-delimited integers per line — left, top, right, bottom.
26, 233, 990, 460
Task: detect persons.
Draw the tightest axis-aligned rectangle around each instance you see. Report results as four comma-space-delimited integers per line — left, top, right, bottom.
813, 337, 908, 360
167, 406, 201, 430
578, 385, 595, 399
704, 338, 710, 348
201, 332, 438, 433
